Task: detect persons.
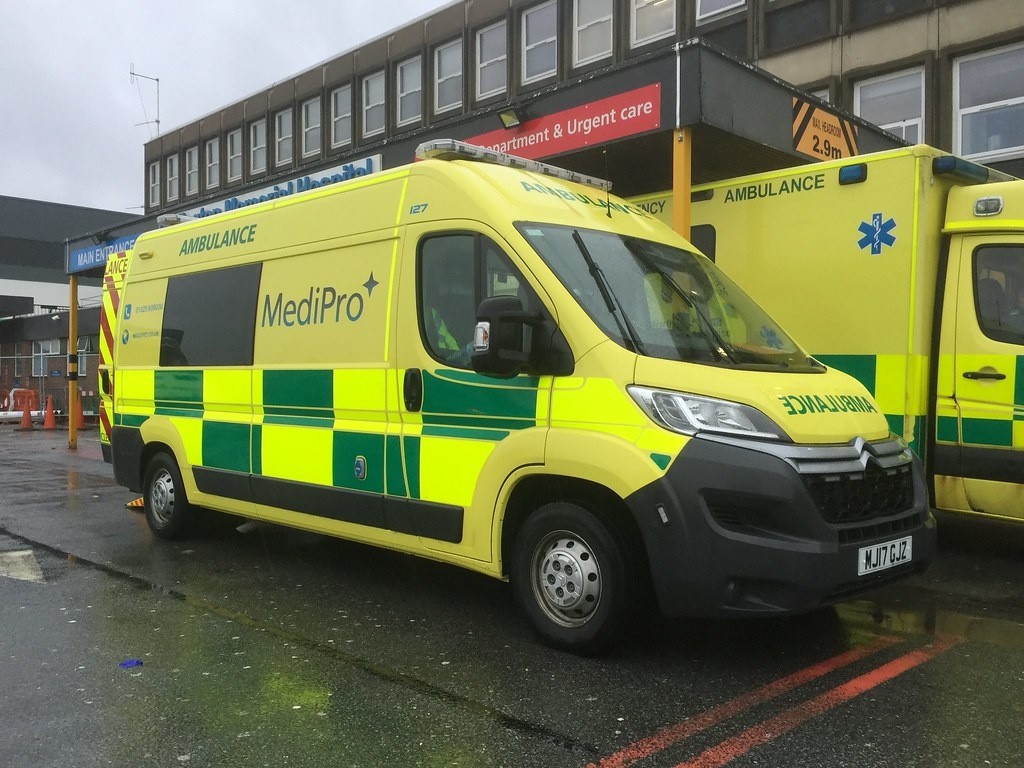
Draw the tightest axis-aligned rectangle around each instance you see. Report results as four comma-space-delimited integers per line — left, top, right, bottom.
542, 270, 645, 352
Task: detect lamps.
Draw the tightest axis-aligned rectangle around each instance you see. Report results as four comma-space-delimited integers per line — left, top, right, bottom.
91, 231, 115, 247
496, 102, 526, 131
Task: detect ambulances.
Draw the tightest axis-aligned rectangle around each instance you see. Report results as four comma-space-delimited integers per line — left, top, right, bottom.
108, 158, 937, 654
617, 144, 1024, 527
